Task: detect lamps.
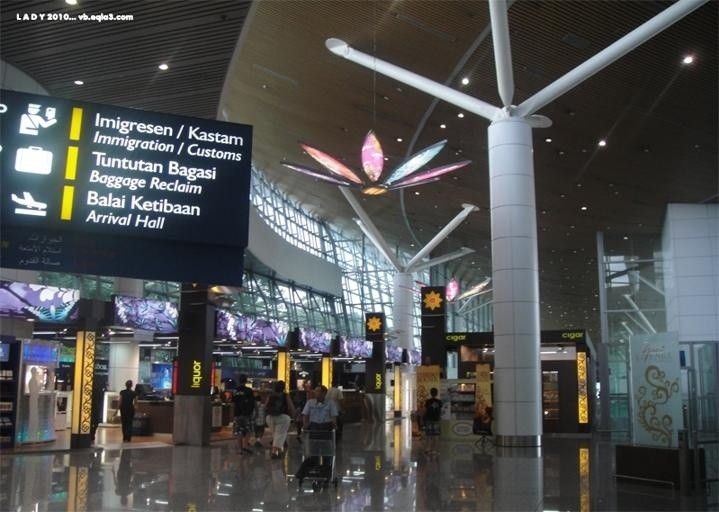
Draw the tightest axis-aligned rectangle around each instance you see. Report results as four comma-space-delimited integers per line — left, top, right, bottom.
279, 1, 472, 197
398, 244, 491, 304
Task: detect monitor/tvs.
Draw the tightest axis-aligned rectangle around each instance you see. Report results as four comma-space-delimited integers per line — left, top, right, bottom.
79, 299, 114, 323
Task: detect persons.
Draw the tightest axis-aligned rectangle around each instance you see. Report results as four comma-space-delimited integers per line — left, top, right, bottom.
118, 380, 138, 442
29, 367, 45, 428
111, 450, 136, 506
230, 375, 346, 483
423, 388, 443, 454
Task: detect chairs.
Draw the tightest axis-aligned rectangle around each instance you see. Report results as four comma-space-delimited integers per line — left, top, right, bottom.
471, 415, 494, 447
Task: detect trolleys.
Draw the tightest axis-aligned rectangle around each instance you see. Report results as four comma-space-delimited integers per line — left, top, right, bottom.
297, 424, 339, 492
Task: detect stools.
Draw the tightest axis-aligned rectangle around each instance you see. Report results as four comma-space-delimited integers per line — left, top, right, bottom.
424, 421, 441, 436
